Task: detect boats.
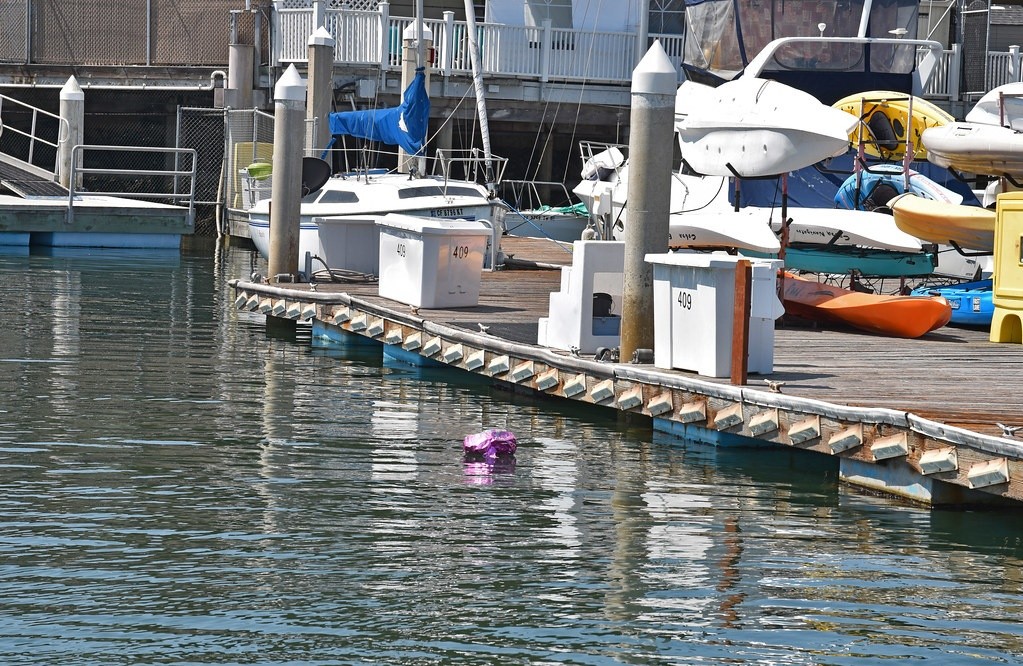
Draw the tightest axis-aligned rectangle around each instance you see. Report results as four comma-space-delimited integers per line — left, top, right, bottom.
238, 0, 511, 276
775, 270, 951, 339
500, 0, 1023, 277
910, 278, 994, 325
